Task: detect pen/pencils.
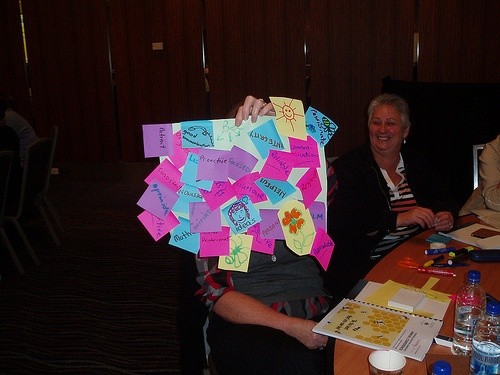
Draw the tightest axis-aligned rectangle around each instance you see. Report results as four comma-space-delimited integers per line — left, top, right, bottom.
431, 263, 469, 267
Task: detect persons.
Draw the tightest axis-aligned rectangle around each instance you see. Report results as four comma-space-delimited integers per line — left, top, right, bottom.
194, 95, 339, 375
0, 105, 23, 197
0, 97, 38, 176
327, 94, 454, 299
457, 134, 500, 216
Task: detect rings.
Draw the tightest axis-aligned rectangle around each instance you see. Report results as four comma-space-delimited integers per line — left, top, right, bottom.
259, 98, 264, 102
319, 344, 323, 350
449, 222, 453, 229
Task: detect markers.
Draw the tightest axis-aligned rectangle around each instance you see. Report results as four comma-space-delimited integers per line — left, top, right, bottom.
425, 247, 456, 255
423, 255, 445, 268
418, 268, 454, 276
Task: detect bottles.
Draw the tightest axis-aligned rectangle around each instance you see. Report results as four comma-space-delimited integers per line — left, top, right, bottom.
451, 270, 487, 356
431, 360, 452, 375
470, 301, 500, 375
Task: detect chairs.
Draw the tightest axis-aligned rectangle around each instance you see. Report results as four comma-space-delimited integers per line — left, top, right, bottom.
2, 124, 66, 249
0, 149, 41, 276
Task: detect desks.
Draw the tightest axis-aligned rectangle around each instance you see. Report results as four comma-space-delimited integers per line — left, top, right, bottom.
326, 212, 500, 375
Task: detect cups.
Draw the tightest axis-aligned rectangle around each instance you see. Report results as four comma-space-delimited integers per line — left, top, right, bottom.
368, 349, 407, 375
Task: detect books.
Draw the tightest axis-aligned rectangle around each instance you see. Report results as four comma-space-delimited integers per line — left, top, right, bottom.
437, 222, 500, 250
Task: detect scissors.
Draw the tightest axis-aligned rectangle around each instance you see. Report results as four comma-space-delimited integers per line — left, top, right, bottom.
396, 255, 421, 268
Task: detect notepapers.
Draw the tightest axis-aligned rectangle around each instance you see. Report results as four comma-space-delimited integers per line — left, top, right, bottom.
388, 287, 426, 313
